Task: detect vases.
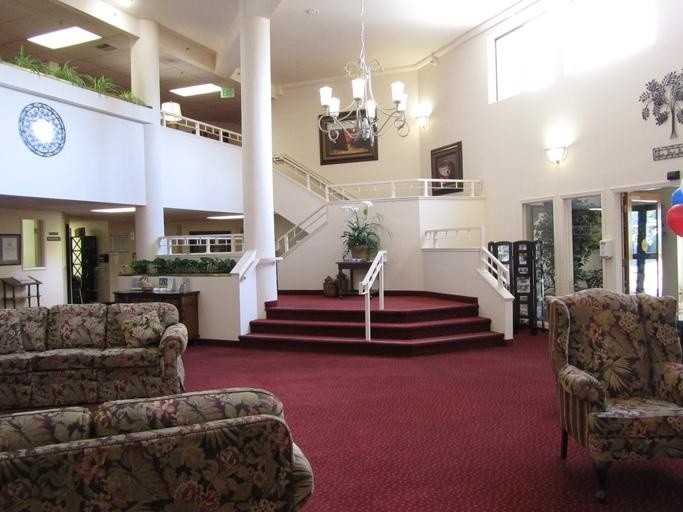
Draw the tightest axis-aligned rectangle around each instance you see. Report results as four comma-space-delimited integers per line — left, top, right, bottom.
350, 244, 368, 261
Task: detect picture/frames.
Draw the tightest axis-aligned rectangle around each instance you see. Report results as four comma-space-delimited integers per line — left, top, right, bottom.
316, 108, 378, 168
429, 139, 463, 197
0, 233, 22, 268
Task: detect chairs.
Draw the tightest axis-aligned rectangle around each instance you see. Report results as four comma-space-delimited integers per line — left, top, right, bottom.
544, 284, 682, 501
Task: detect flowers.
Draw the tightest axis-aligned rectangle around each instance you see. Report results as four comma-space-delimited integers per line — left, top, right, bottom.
335, 200, 391, 257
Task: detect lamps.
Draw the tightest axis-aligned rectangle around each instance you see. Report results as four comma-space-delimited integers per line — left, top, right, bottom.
316, 2, 409, 150
414, 102, 435, 129
543, 146, 568, 166
161, 99, 182, 129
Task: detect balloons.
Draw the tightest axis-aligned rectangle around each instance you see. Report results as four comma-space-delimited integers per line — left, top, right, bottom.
671, 188, 683, 205
667, 203, 683, 237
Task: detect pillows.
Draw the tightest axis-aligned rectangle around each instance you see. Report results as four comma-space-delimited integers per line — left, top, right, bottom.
118, 310, 162, 348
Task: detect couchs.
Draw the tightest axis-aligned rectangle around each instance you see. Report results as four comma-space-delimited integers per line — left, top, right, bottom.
0, 300, 189, 412
0, 385, 315, 511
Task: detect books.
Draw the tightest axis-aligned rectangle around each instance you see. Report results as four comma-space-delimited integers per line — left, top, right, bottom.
13, 274, 36, 284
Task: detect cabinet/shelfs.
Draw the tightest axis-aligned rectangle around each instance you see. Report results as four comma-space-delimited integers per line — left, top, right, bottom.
112, 289, 201, 347
70, 235, 95, 303
0, 274, 43, 309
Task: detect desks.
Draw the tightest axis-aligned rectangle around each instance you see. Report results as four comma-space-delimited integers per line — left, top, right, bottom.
335, 260, 379, 300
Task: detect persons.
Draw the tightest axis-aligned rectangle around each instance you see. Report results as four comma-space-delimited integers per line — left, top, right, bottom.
161, 280, 167, 287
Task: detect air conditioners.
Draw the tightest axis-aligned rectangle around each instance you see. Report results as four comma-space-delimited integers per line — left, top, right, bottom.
93, 252, 109, 303
